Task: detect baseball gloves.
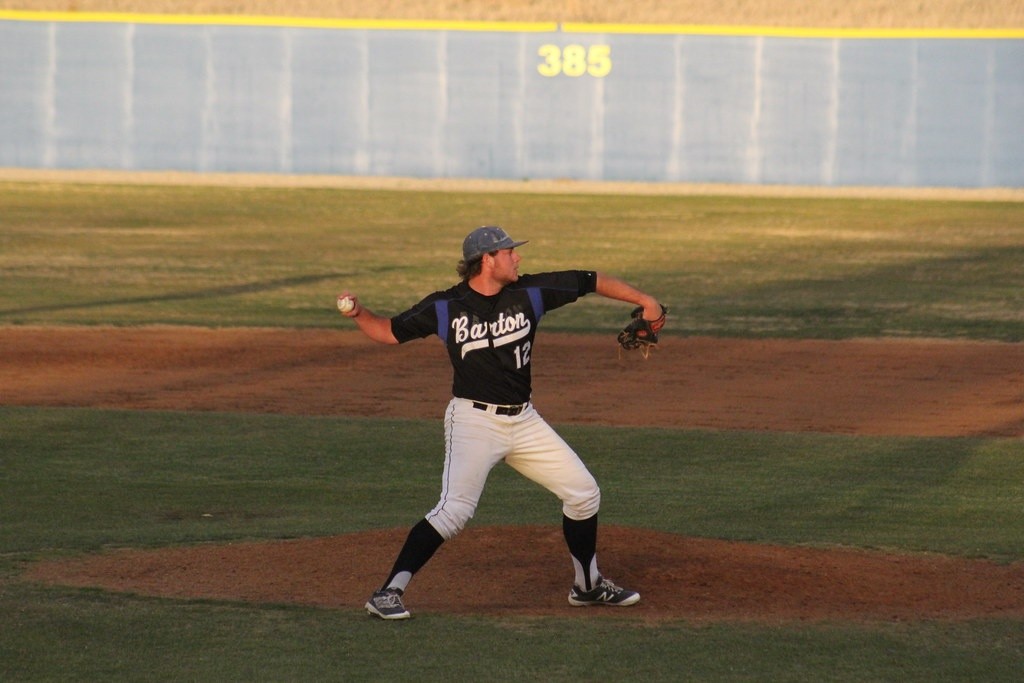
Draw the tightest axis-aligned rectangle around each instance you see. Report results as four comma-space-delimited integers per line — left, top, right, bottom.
616, 305, 666, 352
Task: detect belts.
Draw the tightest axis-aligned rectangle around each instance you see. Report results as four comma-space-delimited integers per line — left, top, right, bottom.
473, 402, 528, 416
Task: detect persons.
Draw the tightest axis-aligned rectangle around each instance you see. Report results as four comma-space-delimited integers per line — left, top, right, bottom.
336, 226, 668, 620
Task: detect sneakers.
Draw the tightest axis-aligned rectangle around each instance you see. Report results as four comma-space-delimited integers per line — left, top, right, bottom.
568, 574, 641, 606
364, 587, 410, 619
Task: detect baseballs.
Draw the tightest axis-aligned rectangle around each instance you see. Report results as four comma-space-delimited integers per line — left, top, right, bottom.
336, 296, 355, 313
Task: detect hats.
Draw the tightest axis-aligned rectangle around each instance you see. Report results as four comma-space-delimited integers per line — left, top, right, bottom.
463, 226, 529, 262
